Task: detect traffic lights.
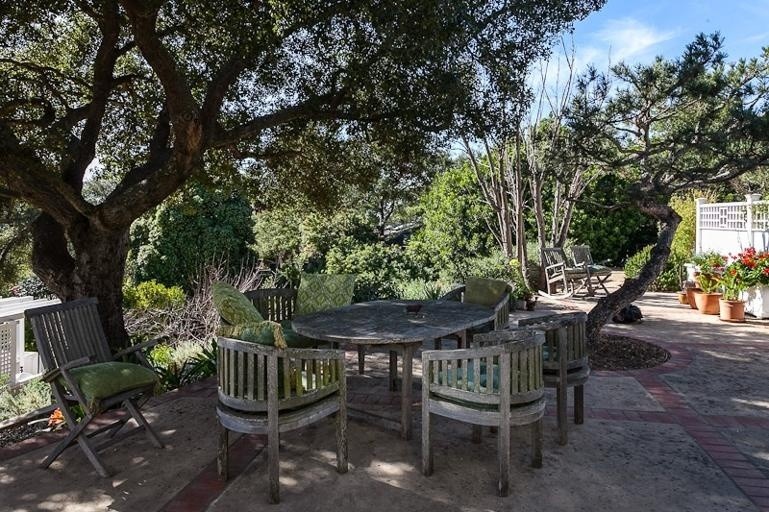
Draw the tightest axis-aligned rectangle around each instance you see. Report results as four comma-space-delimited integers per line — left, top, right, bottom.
292, 274, 355, 317
56, 362, 159, 400
439, 361, 512, 407
217, 321, 301, 411
213, 282, 264, 325
463, 278, 507, 307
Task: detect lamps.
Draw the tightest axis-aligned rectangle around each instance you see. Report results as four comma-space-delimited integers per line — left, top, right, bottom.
421, 330, 545, 496
25, 297, 168, 478
538, 247, 597, 300
216, 336, 348, 504
435, 284, 514, 371
487, 311, 587, 446
571, 246, 611, 297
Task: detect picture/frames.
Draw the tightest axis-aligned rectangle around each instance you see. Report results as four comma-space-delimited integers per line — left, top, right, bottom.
292, 299, 496, 442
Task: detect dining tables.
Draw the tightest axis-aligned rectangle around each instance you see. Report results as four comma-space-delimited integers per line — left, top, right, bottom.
694, 274, 723, 314
719, 276, 745, 322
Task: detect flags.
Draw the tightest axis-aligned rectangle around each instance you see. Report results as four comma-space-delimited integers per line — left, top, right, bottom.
693, 272, 700, 287
679, 293, 686, 304
686, 288, 703, 309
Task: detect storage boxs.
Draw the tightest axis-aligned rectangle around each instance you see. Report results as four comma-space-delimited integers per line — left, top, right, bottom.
221, 288, 364, 376
